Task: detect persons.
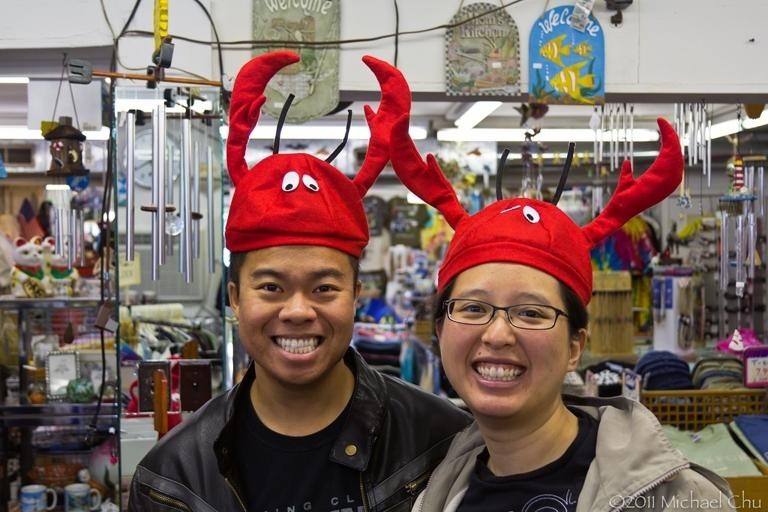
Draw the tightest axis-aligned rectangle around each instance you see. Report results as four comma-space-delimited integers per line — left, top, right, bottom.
411, 262, 737, 512
128, 245, 475, 511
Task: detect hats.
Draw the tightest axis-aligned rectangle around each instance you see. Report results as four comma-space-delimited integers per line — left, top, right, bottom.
389, 112, 685, 308
226, 52, 413, 257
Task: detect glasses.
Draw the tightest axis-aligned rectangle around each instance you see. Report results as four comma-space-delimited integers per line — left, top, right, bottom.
441, 298, 570, 330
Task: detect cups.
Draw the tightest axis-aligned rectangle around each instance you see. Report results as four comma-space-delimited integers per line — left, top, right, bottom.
63, 482, 102, 512
20, 484, 57, 512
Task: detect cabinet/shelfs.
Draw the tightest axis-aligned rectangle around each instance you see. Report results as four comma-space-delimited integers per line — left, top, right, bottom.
0, 298, 121, 512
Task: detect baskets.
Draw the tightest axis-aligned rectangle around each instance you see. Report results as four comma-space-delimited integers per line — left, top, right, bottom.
640, 364, 768, 434
